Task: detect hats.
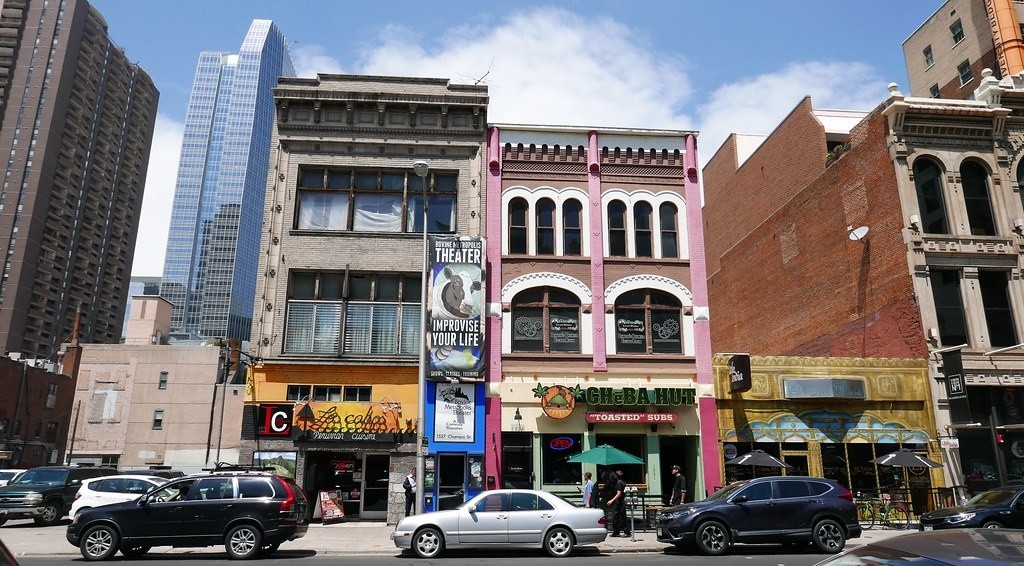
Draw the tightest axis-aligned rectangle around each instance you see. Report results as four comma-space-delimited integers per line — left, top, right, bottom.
670, 465, 681, 471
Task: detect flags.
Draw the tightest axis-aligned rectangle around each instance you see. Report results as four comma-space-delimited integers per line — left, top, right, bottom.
941, 348, 968, 400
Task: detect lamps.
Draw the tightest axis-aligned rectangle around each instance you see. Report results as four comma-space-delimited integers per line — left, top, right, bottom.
514, 406, 522, 420
381, 396, 398, 410
292, 395, 315, 411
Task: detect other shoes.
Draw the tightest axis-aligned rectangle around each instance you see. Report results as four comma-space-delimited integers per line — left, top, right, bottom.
610, 534, 620, 537
621, 534, 632, 538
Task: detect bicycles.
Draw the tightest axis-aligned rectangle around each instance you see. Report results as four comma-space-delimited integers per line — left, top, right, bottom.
856, 499, 909, 530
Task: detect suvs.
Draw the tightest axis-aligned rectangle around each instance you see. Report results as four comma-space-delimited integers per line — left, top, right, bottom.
65, 462, 312, 560
656, 476, 863, 556
0, 465, 119, 527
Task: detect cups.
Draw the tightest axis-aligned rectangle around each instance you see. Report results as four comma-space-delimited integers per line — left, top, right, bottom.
857, 490, 861, 498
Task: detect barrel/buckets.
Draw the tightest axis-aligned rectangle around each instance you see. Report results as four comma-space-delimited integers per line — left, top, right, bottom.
646, 510, 657, 528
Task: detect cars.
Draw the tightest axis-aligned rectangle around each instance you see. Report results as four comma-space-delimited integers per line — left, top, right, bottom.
67, 475, 179, 522
125, 469, 184, 481
0, 469, 28, 488
389, 489, 609, 559
811, 528, 1023, 565
917, 483, 1024, 533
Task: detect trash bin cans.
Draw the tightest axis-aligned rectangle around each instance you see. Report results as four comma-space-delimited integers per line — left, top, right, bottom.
909, 480, 929, 515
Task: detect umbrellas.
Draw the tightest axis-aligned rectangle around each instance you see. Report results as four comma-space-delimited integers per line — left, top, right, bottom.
868, 448, 945, 519
566, 444, 646, 532
724, 449, 793, 479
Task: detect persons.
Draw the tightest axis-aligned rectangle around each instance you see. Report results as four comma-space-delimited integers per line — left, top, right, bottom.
403, 467, 416, 516
669, 465, 686, 505
580, 472, 595, 508
606, 472, 632, 537
177, 486, 190, 500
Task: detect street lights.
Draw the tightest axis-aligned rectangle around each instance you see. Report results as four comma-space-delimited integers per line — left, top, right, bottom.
411, 161, 430, 517
